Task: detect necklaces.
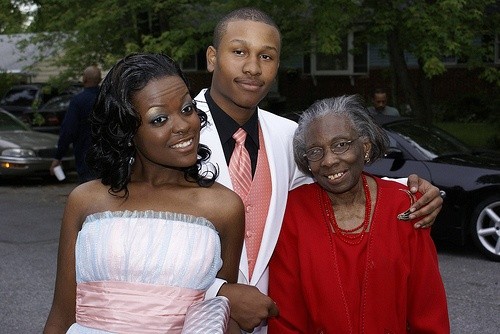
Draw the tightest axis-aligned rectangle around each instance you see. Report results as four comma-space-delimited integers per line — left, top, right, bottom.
325, 176, 372, 245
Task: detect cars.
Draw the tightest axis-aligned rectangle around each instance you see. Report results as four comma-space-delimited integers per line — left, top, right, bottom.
274, 110, 500, 263
0, 77, 85, 133
0, 106, 83, 186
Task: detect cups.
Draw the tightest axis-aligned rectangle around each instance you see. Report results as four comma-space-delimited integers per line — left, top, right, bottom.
53, 165, 66, 181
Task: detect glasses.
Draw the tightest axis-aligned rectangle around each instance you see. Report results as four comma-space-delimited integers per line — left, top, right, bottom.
302, 139, 355, 162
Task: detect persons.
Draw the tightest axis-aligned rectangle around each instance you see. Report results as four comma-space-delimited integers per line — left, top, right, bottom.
50, 65, 102, 183
366, 88, 400, 117
267, 94, 450, 334
42, 54, 245, 334
192, 8, 443, 334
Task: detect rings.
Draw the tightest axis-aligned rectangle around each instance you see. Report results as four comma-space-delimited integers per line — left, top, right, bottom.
440, 190, 446, 199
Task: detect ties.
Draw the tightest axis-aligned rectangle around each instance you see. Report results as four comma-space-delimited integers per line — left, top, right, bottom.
228, 128, 252, 201
